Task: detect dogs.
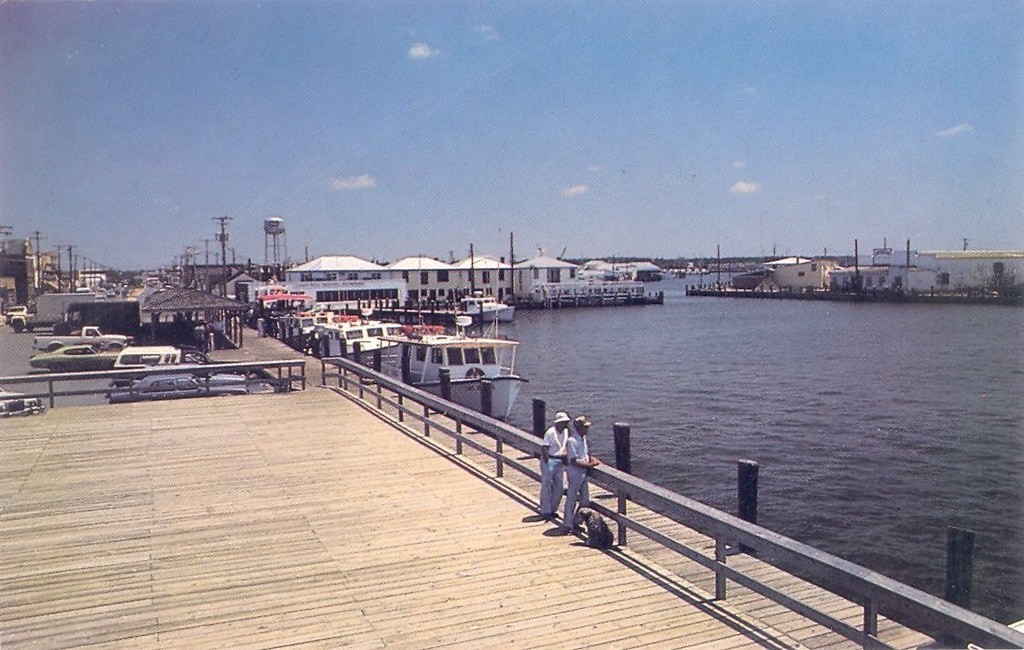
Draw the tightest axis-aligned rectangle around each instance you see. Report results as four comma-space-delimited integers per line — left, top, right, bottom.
577, 507, 614, 550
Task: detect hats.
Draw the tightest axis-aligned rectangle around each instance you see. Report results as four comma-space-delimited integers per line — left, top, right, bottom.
553, 411, 570, 423
573, 415, 592, 426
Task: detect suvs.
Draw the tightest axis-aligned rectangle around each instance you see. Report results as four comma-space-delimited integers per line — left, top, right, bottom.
113, 344, 213, 378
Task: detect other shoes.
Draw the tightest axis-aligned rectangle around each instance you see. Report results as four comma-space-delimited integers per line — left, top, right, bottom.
560, 526, 575, 533
542, 513, 559, 519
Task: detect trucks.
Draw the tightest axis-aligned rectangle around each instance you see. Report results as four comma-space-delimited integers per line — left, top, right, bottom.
53, 300, 142, 335
12, 291, 98, 331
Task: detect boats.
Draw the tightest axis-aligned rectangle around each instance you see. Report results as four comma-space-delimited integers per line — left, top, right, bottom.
253, 231, 532, 421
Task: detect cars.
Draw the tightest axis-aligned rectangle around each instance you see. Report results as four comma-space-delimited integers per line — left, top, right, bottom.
6, 307, 36, 319
0, 388, 47, 414
28, 345, 122, 373
110, 368, 253, 404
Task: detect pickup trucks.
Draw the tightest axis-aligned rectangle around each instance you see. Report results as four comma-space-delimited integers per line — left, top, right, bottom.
35, 327, 130, 349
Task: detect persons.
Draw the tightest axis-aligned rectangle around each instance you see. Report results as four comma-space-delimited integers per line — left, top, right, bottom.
194, 319, 214, 354
257, 315, 265, 338
566, 416, 601, 531
540, 412, 570, 520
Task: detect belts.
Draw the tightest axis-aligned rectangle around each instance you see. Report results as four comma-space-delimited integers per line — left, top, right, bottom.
549, 455, 562, 459
